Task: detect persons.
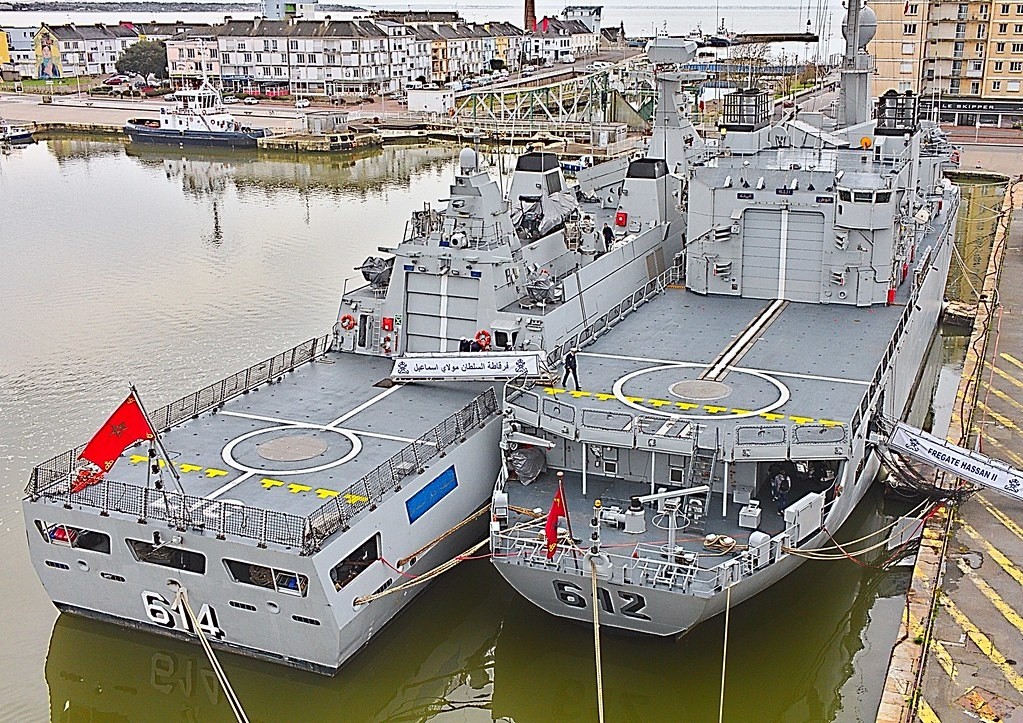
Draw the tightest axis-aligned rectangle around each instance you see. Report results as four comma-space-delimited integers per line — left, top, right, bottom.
459, 336, 481, 352
768, 462, 793, 516
603, 222, 615, 248
562, 347, 582, 389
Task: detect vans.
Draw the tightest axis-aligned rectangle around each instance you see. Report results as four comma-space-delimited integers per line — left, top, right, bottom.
563, 55, 576, 63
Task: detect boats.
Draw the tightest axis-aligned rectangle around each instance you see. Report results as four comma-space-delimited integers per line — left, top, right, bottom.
256, 109, 385, 155
0, 116, 37, 145
123, 38, 262, 146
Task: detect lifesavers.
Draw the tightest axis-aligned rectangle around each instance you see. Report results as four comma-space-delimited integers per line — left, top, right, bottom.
475, 330, 491, 346
341, 314, 354, 330
202, 110, 206, 115
211, 120, 215, 124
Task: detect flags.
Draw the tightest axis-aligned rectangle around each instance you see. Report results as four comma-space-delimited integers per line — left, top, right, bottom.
78, 393, 154, 474
544, 487, 566, 561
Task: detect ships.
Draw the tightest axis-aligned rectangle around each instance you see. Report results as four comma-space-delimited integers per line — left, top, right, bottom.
486, 0, 964, 644
16, 63, 709, 679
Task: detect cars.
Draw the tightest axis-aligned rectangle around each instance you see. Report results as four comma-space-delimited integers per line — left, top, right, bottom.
243, 97, 260, 105
389, 70, 509, 105
223, 96, 240, 104
521, 62, 554, 77
586, 61, 614, 71
294, 99, 311, 109
103, 77, 122, 85
114, 75, 129, 82
